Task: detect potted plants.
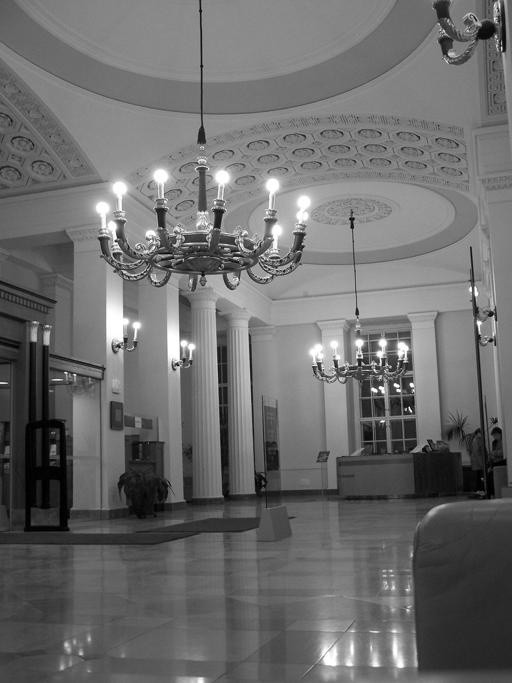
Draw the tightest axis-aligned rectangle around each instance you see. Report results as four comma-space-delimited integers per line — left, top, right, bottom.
117, 468, 175, 518
445, 411, 475, 490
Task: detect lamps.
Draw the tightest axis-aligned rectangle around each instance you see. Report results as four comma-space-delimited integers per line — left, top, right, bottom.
476, 320, 496, 346
172, 341, 196, 370
469, 285, 497, 322
112, 319, 141, 354
94, 0, 311, 291
310, 210, 410, 383
371, 383, 414, 413
432, 0, 506, 65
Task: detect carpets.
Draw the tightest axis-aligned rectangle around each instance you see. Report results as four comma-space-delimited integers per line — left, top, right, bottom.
136, 517, 295, 532
0, 532, 199, 545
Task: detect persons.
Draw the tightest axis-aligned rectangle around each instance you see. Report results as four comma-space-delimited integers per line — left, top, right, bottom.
471, 427, 503, 496
